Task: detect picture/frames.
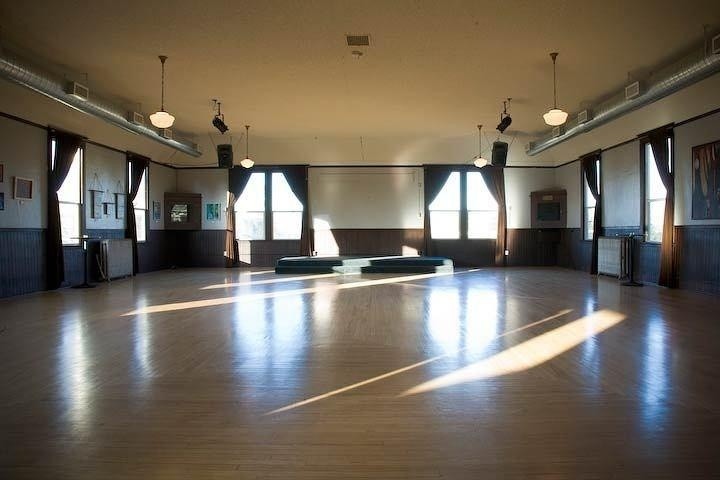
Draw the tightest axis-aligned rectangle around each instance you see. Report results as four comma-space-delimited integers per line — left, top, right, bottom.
691, 140, 720, 220
0, 163, 33, 211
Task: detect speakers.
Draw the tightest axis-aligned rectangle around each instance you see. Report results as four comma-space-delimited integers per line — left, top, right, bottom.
217, 144, 233, 168
491, 141, 508, 167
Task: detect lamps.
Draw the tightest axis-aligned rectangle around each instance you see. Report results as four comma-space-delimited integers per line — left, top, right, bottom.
544, 51, 569, 127
149, 55, 175, 129
239, 126, 255, 168
474, 124, 487, 168
210, 99, 229, 135
495, 99, 511, 134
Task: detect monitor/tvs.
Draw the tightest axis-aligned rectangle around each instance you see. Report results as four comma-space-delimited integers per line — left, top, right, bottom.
168, 200, 192, 224
537, 202, 560, 221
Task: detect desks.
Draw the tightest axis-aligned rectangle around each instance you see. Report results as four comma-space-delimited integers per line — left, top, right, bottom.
619, 234, 644, 286
70, 237, 100, 288
